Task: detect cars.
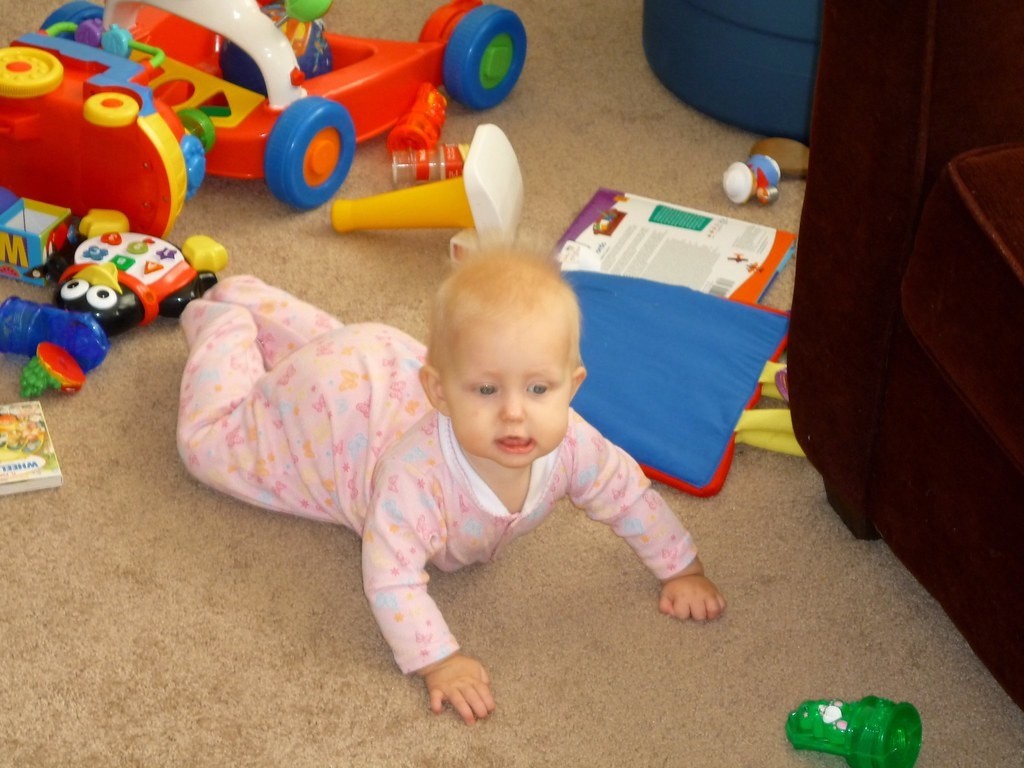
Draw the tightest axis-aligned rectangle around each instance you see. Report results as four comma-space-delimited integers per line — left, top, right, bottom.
0, 32, 214, 242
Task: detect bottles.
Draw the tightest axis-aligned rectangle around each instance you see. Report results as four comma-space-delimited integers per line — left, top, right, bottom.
392, 144, 471, 187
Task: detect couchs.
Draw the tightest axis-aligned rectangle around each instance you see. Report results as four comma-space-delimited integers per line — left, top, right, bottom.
787, 0, 1024, 715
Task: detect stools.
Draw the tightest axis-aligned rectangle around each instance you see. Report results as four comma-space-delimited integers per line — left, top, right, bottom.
642, 0, 823, 141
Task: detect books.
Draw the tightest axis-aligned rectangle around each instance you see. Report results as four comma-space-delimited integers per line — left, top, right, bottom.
548, 186, 798, 304
0, 400, 63, 496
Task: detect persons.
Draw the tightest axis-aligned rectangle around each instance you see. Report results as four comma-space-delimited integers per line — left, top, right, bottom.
177, 238, 726, 726
